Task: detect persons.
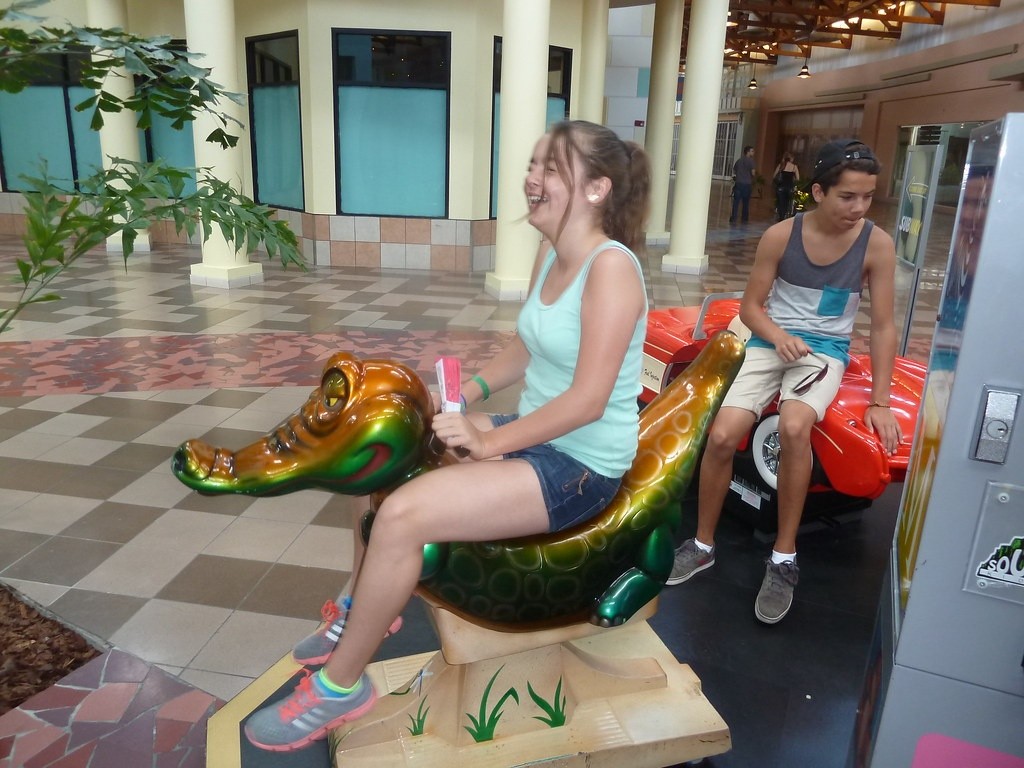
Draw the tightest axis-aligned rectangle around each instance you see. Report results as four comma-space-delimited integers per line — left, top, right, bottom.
664, 138, 904, 622
244, 120, 652, 752
730, 146, 756, 223
773, 153, 799, 220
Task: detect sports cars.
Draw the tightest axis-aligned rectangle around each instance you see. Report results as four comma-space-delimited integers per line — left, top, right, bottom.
640, 289, 931, 506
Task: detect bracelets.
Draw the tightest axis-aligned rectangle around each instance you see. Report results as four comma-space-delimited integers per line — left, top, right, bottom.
869, 401, 892, 407
459, 394, 466, 413
471, 375, 489, 401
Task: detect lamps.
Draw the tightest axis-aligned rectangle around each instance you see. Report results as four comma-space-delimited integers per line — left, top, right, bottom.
746, 44, 759, 91
795, 33, 812, 78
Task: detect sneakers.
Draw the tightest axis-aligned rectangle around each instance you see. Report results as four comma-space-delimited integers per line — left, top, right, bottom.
293, 595, 403, 665
244, 668, 377, 751
754, 550, 799, 625
664, 537, 714, 586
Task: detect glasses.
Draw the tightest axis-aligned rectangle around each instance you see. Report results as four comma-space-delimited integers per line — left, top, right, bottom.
791, 352, 829, 396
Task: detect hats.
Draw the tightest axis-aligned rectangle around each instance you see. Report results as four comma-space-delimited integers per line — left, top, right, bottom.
802, 139, 875, 193
